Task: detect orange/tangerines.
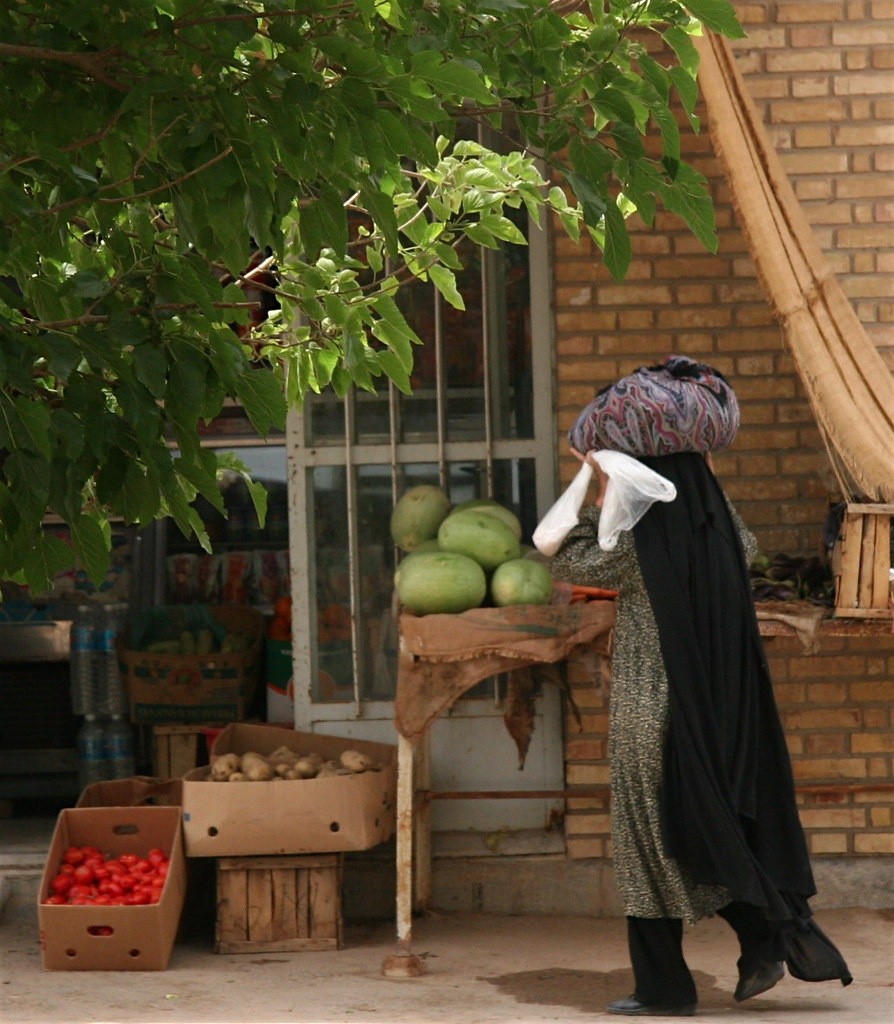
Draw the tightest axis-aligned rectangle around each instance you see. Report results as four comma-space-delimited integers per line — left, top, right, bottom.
270, 596, 352, 645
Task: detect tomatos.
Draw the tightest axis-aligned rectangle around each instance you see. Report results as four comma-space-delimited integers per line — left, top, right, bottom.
44, 843, 167, 935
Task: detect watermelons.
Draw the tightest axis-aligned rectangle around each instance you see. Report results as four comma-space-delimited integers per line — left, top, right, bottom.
391, 484, 554, 613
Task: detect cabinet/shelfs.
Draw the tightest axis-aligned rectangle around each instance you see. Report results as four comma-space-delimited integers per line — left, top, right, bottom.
150, 385, 523, 610
0, 507, 146, 801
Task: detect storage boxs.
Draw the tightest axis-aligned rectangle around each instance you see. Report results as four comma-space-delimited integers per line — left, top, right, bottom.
35, 803, 186, 975
116, 602, 266, 726
75, 774, 184, 808
182, 721, 394, 858
831, 502, 894, 621
213, 852, 346, 956
266, 636, 353, 727
151, 726, 219, 777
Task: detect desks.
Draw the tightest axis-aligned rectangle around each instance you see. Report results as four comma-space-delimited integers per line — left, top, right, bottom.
380, 599, 894, 982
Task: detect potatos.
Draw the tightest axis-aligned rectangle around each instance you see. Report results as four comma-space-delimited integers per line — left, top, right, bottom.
201, 748, 381, 782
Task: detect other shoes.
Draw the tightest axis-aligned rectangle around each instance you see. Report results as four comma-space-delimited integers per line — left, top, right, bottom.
734, 952, 785, 1004
606, 993, 696, 1017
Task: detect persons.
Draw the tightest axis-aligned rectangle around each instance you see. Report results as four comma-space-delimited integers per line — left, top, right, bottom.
547, 442, 852, 1018
